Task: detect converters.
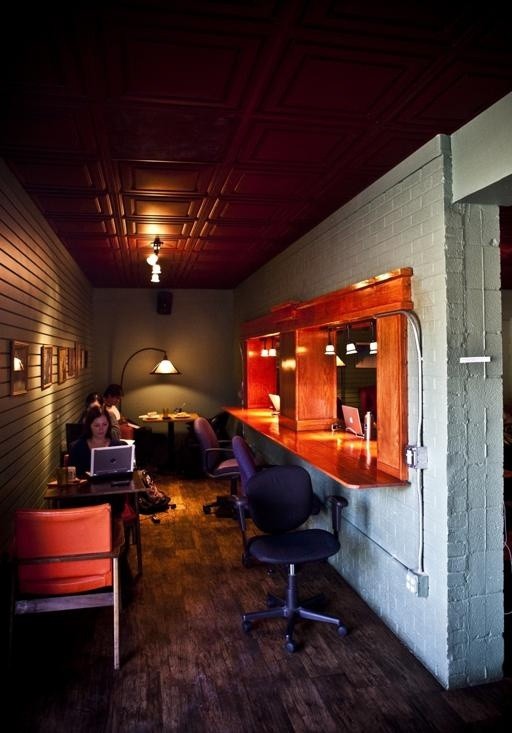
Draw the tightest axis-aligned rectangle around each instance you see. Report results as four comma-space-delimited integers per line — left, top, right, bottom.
153, 516, 160, 523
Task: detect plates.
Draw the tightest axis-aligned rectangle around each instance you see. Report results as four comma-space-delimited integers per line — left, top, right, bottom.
173, 414, 190, 418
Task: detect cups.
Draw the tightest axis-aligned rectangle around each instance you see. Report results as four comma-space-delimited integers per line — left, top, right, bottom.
55, 466, 76, 488
163, 408, 168, 418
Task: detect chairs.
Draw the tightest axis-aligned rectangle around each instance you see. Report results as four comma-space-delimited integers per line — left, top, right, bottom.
185, 411, 349, 653
13, 502, 127, 671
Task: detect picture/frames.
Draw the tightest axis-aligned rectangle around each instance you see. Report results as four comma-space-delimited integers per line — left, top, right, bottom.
10, 338, 84, 396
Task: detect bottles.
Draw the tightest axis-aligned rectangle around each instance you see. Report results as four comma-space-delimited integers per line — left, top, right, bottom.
364, 411, 374, 440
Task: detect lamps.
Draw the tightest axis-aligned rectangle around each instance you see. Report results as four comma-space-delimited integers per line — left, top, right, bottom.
118, 346, 182, 413
322, 319, 377, 356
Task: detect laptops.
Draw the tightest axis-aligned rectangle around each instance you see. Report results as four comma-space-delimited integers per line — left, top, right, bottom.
85, 444, 135, 478
342, 405, 363, 436
268, 393, 281, 412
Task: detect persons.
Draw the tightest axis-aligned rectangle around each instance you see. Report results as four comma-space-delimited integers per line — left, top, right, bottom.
65, 403, 130, 519
77, 390, 122, 443
103, 382, 129, 431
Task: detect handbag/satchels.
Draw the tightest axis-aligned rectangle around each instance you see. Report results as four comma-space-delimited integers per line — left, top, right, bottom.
133, 469, 170, 513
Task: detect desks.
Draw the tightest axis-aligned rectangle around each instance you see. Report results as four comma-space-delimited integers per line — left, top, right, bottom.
44, 471, 149, 576
142, 411, 199, 463
